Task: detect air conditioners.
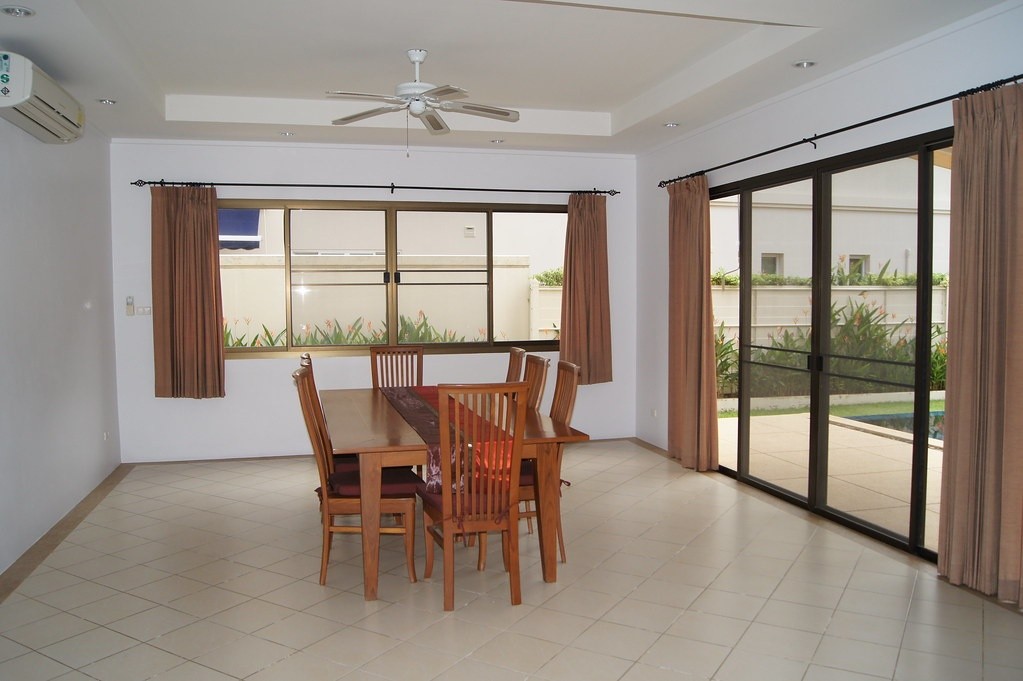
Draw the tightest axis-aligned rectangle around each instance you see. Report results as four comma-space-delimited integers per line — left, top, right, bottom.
0, 50, 85, 145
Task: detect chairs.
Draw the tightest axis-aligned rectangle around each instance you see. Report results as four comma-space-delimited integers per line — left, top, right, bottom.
292, 344, 581, 610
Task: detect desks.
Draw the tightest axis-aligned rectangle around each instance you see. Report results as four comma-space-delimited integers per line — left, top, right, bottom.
318, 385, 590, 601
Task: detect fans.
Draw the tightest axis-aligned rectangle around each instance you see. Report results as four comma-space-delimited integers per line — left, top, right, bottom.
324, 49, 519, 136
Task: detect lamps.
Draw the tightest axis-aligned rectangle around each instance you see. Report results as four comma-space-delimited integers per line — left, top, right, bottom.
406, 50, 428, 116
